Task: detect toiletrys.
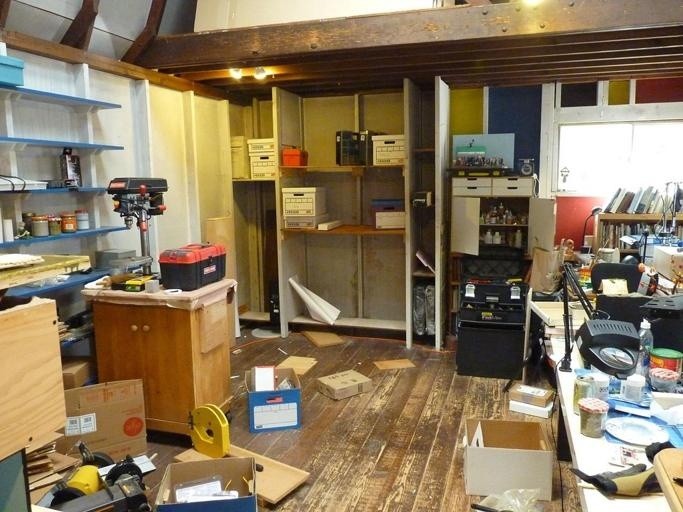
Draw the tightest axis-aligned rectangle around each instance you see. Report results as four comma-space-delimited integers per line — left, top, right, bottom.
479, 227, 523, 249
480, 200, 528, 226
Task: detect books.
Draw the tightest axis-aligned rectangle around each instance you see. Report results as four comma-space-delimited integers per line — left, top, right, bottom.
598, 220, 683, 251
602, 185, 682, 214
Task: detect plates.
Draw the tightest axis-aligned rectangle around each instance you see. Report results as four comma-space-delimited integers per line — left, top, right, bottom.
605, 416, 669, 446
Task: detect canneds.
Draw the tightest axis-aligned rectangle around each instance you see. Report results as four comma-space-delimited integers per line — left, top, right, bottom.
30, 217, 49, 237
75, 208, 89, 230
61, 213, 77, 233
578, 397, 610, 438
22, 212, 35, 231
49, 217, 63, 236
573, 375, 594, 416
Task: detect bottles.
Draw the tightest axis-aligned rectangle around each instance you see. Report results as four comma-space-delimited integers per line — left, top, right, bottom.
514, 230, 522, 248
479, 202, 517, 224
636, 319, 654, 377
484, 228, 501, 244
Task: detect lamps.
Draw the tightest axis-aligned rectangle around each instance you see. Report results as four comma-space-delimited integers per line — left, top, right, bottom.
581, 207, 602, 247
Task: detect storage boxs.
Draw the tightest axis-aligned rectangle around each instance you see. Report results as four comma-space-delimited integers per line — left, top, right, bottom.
248, 152, 275, 179
158, 243, 226, 289
461, 417, 554, 502
244, 367, 301, 432
282, 186, 326, 216
370, 134, 405, 164
155, 457, 258, 512
283, 215, 330, 230
375, 212, 406, 230
228, 134, 249, 180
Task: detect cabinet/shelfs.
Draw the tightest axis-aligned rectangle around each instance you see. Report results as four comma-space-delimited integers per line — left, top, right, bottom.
594, 210, 683, 257
0, 81, 140, 351
451, 173, 557, 257
93, 290, 234, 441
273, 78, 415, 350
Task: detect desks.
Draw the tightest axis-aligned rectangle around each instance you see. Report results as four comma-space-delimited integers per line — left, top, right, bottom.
549, 337, 674, 512
